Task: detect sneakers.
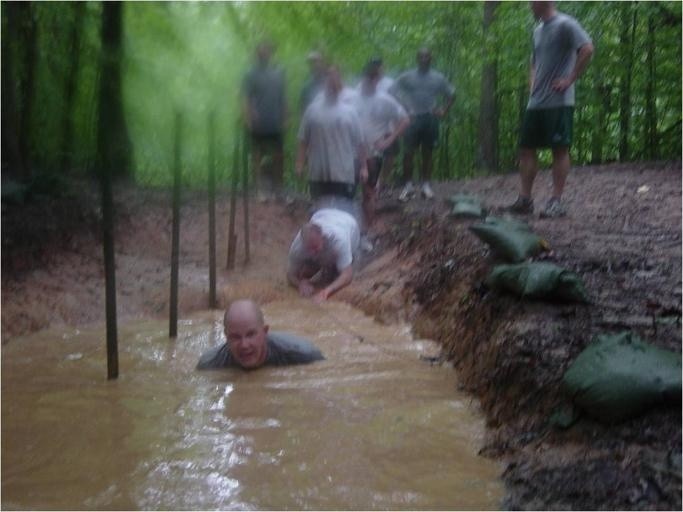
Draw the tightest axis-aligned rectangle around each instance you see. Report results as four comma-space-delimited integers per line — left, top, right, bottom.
539, 198, 566, 218
397, 184, 436, 203
498, 196, 534, 215
252, 187, 295, 206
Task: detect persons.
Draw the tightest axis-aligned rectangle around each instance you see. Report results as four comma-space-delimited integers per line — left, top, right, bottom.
294, 65, 370, 200
297, 52, 346, 114
285, 193, 367, 308
495, 2, 594, 220
353, 62, 410, 253
194, 299, 325, 372
239, 37, 288, 204
356, 53, 396, 198
384, 47, 456, 204
